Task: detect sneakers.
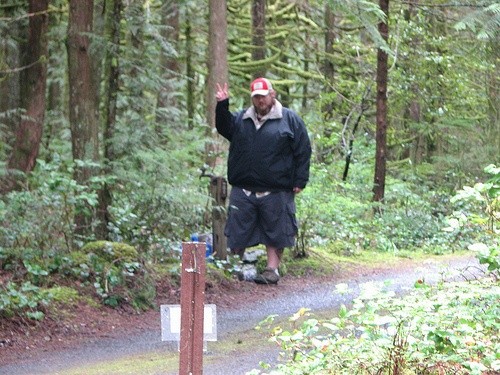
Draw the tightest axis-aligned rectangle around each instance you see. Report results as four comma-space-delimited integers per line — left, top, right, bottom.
254, 266, 282, 284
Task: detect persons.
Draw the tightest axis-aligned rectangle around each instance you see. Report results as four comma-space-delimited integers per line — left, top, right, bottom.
215, 77, 311, 285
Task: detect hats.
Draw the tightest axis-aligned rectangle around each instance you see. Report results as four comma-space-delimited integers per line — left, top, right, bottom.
250, 78, 273, 98
